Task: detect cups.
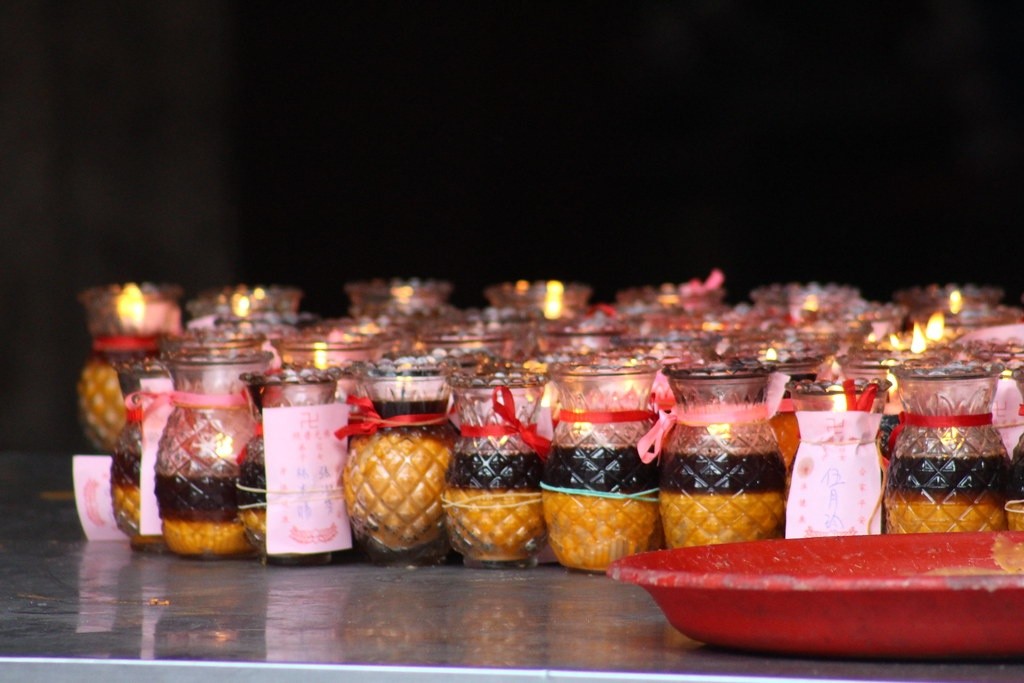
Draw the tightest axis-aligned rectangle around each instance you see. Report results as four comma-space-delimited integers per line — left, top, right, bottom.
75, 282, 1024, 571
